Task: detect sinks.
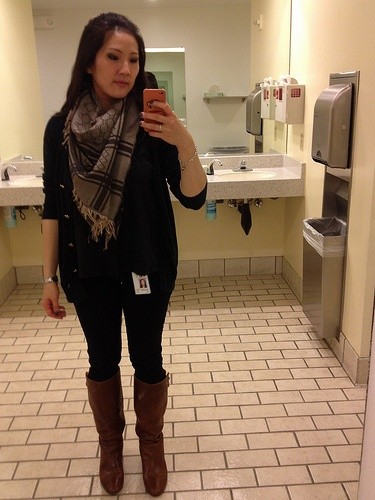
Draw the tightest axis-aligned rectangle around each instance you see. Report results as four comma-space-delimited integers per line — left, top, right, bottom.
7, 177, 44, 186
218, 171, 277, 181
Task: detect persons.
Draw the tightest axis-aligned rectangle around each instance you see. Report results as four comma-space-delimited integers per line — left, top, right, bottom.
144, 71, 159, 90
39, 10, 207, 497
140, 279, 147, 288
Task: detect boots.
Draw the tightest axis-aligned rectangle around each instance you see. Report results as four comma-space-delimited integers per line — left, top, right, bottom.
86, 367, 125, 494
134, 371, 169, 497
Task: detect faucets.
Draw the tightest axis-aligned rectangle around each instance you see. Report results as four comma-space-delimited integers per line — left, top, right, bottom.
208, 159, 223, 175
19, 156, 32, 162
205, 151, 216, 155
40, 165, 44, 177
238, 159, 248, 172
1, 164, 17, 181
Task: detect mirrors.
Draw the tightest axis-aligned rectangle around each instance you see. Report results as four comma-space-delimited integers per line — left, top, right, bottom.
0, 0, 375, 500
0, 0, 290, 166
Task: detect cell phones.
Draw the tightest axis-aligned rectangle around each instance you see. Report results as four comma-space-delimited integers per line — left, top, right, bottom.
144, 89, 166, 133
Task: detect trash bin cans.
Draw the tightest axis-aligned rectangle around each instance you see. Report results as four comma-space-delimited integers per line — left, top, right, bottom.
302, 217, 347, 338
209, 146, 246, 152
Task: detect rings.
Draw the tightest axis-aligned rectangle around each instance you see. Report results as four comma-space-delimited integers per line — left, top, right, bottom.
159, 124, 162, 132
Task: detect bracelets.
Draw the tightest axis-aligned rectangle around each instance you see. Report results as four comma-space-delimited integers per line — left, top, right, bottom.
178, 145, 198, 171
42, 276, 58, 284
